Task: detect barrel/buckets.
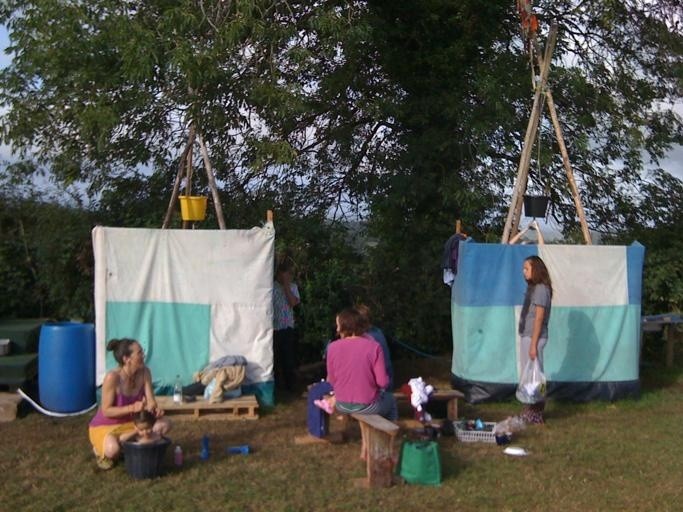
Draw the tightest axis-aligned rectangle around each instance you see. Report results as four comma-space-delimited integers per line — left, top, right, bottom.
177, 195, 209, 221
38, 318, 96, 413
121, 436, 172, 480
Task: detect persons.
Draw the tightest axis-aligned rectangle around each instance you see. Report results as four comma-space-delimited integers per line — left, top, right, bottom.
120, 410, 162, 444
314, 334, 376, 415
274, 264, 301, 392
326, 309, 399, 465
519, 256, 552, 423
352, 304, 394, 392
89, 339, 173, 470
508, 221, 544, 245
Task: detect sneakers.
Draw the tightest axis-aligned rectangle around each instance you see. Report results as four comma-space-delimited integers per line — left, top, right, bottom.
97, 454, 114, 470
312, 399, 334, 414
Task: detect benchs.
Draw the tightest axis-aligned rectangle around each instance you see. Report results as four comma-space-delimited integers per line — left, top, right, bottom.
295, 379, 467, 492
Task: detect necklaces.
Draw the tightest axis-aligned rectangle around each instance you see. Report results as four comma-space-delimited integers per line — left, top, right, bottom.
122, 369, 137, 388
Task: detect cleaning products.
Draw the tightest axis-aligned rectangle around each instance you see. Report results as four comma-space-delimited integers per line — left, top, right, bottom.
174, 443, 183, 467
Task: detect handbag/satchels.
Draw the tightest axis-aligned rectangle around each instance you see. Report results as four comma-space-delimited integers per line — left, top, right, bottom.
395, 438, 442, 484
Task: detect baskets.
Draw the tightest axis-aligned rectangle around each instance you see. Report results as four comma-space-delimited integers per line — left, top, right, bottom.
452, 420, 497, 443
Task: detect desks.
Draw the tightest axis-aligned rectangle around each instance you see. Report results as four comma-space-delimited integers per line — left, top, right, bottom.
131, 387, 261, 426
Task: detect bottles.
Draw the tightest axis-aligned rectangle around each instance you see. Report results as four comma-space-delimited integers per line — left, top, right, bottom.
171, 445, 185, 468
200, 432, 209, 461
173, 375, 183, 405
